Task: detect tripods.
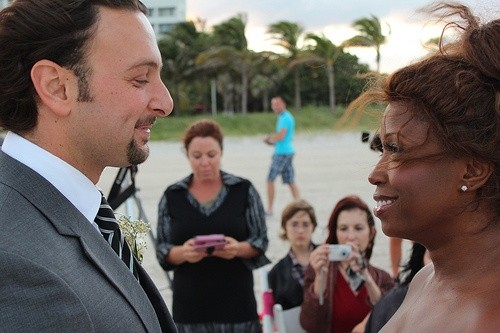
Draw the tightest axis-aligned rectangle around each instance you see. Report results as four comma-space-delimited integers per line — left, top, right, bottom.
104, 163, 175, 291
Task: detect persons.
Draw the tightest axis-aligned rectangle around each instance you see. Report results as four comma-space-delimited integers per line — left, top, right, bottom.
350, 240, 433, 333
154, 119, 274, 333
263, 95, 303, 217
333, 1, 500, 333
267, 199, 330, 313
298, 194, 400, 333
0, 1, 177, 333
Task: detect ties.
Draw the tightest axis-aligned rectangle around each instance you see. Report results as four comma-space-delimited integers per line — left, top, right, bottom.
94, 195, 140, 284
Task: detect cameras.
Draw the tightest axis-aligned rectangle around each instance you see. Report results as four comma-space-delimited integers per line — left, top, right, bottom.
327, 244, 352, 262
192, 234, 227, 255
